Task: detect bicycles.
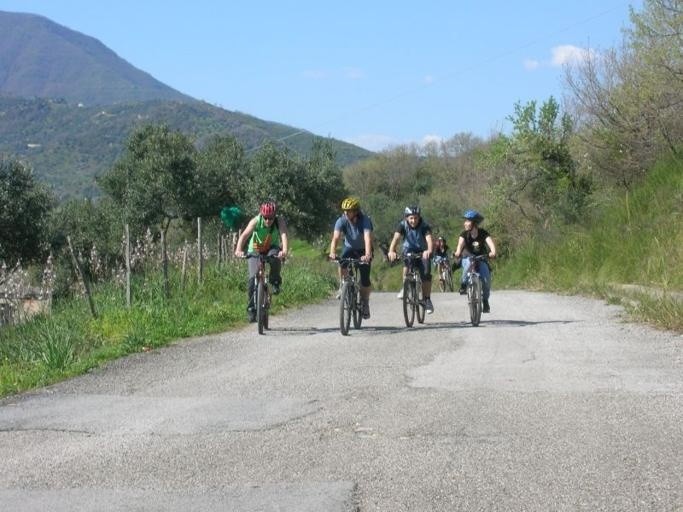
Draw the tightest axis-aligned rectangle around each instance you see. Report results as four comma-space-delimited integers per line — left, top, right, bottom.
328, 258, 362, 336
237, 255, 287, 335
435, 256, 453, 293
389, 254, 430, 326
457, 253, 493, 326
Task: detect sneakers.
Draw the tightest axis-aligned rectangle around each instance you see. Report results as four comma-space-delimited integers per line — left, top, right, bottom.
362, 304, 370, 319
397, 288, 409, 299
459, 283, 467, 294
483, 300, 490, 312
247, 306, 256, 322
335, 287, 344, 299
273, 280, 280, 295
423, 297, 433, 314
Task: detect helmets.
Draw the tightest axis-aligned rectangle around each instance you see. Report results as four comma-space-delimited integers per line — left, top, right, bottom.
461, 209, 484, 224
341, 198, 360, 211
404, 204, 420, 217
260, 202, 276, 218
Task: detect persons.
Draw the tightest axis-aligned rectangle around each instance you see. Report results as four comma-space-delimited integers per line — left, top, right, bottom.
329, 198, 373, 318
236, 202, 288, 324
387, 206, 434, 315
433, 236, 453, 281
454, 209, 496, 312
